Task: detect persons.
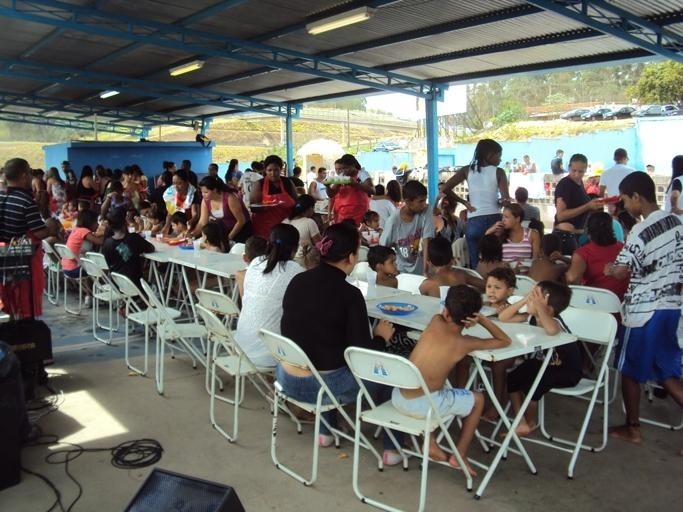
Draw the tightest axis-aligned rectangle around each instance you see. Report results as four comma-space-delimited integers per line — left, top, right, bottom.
387, 282, 512, 478
439, 138, 511, 270
240, 151, 485, 298
233, 222, 315, 422
0, 158, 254, 320
455, 265, 517, 421
494, 279, 583, 440
501, 147, 683, 355
601, 168, 683, 445
271, 221, 416, 469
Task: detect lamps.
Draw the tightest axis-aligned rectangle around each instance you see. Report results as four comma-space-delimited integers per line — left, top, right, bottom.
165, 57, 207, 78
303, 4, 378, 36
97, 86, 120, 100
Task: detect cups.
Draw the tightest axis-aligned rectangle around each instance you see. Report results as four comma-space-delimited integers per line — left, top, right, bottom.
58, 214, 63, 220
192, 241, 200, 252
144, 231, 150, 241
439, 286, 450, 300
367, 271, 376, 288
128, 227, 134, 233
155, 231, 162, 242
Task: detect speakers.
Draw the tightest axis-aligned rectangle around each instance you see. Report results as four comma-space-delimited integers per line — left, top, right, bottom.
124, 468, 246, 512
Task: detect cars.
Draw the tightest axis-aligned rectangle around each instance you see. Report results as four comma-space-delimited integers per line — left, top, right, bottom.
560, 104, 679, 120
372, 142, 400, 152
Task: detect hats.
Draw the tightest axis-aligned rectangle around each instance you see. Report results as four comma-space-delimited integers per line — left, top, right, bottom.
61, 160, 69, 165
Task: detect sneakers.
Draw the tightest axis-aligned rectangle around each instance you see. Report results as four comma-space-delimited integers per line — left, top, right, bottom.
85, 296, 94, 306
383, 447, 413, 466
317, 425, 349, 449
119, 305, 127, 318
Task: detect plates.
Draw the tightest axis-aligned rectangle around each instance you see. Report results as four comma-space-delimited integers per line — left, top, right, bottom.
376, 302, 417, 316
595, 197, 623, 205
247, 200, 286, 208
178, 244, 193, 249
